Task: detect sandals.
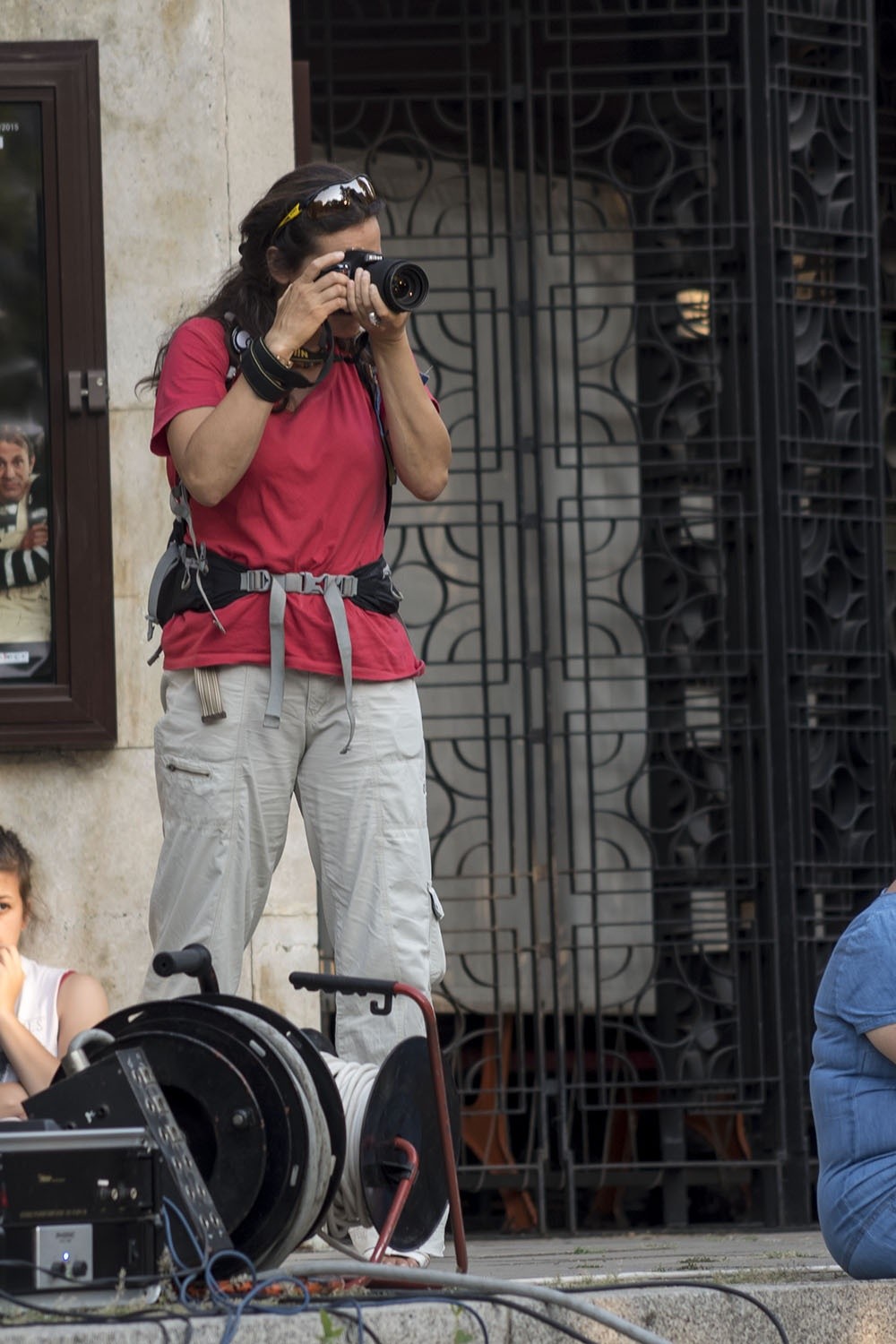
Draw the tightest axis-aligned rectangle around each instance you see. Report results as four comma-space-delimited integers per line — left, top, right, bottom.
364, 1248, 430, 1268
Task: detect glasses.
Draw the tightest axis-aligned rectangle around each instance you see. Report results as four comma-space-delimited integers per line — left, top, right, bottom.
269, 173, 377, 246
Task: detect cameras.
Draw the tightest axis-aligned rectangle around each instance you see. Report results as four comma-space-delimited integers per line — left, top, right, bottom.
322, 247, 430, 311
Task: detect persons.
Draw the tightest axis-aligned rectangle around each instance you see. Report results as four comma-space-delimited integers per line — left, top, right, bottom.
0, 825, 107, 1126
809, 880, 896, 1277
138, 160, 451, 1279
0, 423, 49, 642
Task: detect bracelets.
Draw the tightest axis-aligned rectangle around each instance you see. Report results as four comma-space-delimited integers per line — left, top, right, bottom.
243, 338, 304, 402
